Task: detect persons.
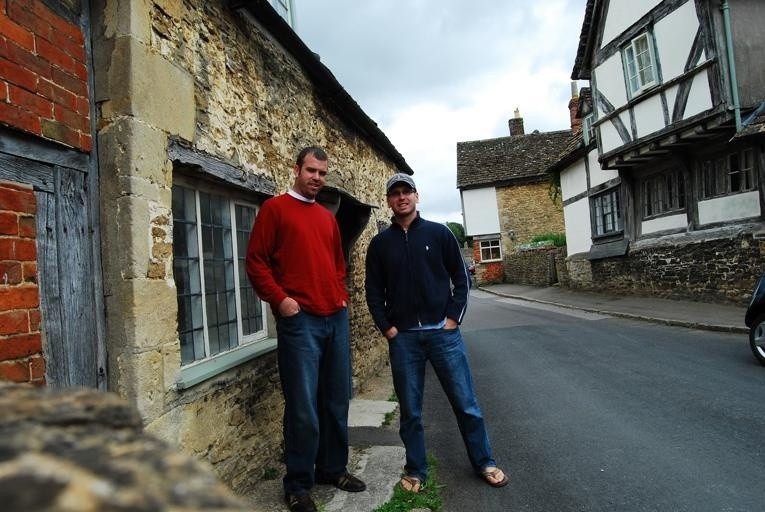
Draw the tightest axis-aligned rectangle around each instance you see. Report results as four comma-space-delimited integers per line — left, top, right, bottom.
245, 147, 366, 512
365, 173, 509, 494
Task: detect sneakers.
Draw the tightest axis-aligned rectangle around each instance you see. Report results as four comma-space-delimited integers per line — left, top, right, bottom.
284, 494, 317, 511
314, 469, 365, 491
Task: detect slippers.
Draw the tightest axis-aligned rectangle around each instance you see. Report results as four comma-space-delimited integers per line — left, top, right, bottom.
482, 465, 508, 486
401, 476, 421, 494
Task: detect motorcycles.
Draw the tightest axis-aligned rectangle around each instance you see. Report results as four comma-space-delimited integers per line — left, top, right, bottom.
744, 267, 765, 361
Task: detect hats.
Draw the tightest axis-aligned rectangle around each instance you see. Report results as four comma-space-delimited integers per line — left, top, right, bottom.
387, 174, 415, 192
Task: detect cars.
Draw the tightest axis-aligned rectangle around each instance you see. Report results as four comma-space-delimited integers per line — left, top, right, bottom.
467, 259, 475, 275
